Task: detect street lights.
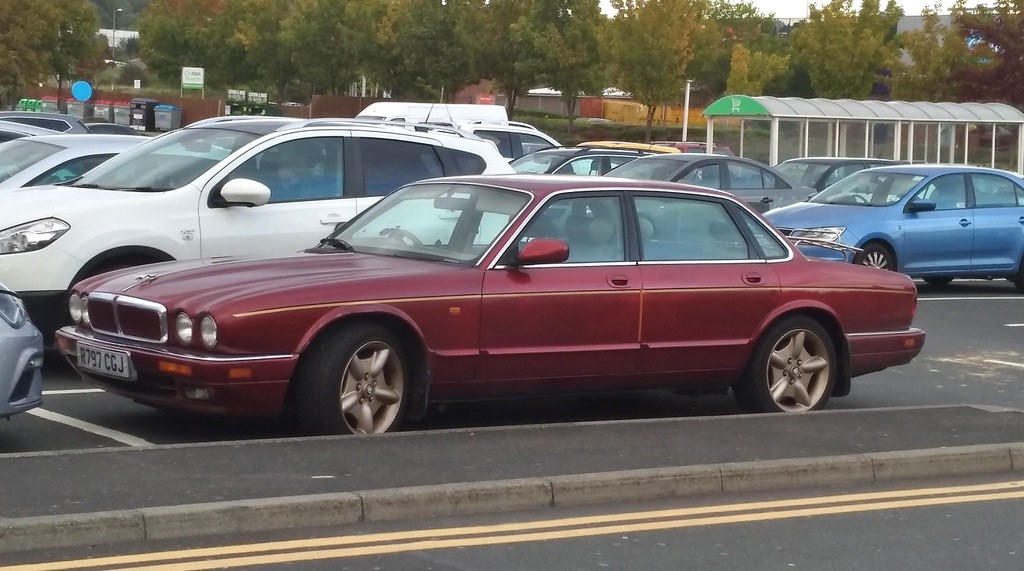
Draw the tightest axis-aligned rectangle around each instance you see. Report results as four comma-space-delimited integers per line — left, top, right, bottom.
111, 9, 122, 91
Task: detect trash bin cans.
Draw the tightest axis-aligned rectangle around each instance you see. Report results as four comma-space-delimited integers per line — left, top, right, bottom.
17, 98, 42, 112
154, 104, 183, 132
225, 99, 285, 116
131, 98, 158, 133
112, 101, 131, 126
39, 100, 59, 113
67, 101, 91, 124
93, 101, 113, 123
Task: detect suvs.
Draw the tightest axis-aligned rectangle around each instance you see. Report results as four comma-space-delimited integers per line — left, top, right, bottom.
0, 114, 537, 380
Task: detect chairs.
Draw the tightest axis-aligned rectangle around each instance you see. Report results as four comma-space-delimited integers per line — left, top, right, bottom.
589, 159, 599, 175
508, 213, 557, 242
565, 212, 655, 261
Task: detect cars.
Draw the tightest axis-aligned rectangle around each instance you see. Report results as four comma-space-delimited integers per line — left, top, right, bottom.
1, 133, 232, 221
0, 283, 46, 419
54, 173, 928, 438
0, 102, 943, 306
746, 163, 1024, 295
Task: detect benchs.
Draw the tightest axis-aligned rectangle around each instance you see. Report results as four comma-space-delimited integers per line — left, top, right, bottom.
637, 212, 746, 259
